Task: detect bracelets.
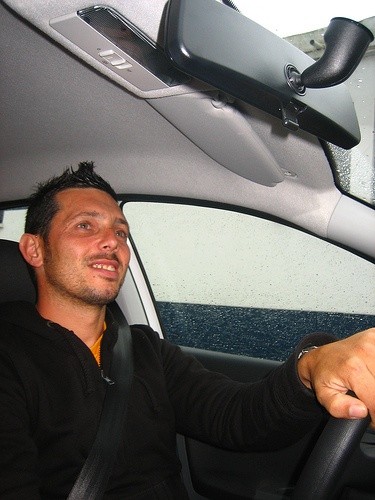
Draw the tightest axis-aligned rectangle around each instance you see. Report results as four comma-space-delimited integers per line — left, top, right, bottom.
297, 343, 319, 360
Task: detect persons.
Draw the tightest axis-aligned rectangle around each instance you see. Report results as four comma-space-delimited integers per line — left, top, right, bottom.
0, 161, 375, 500
